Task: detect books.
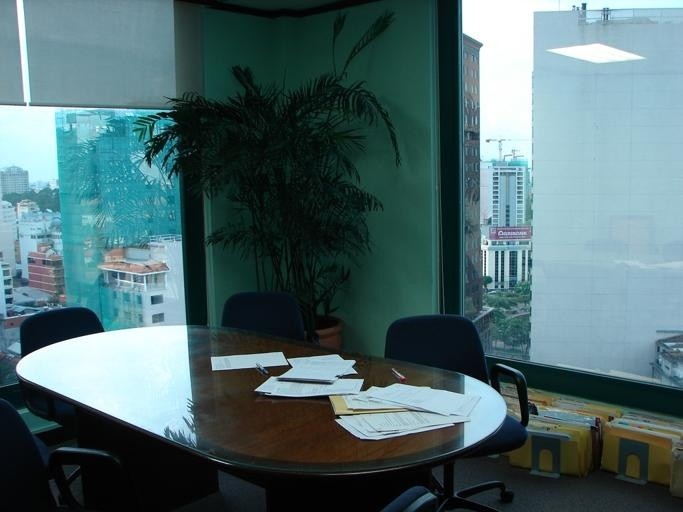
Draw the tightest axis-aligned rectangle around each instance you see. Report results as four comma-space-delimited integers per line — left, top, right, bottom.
502, 380, 679, 489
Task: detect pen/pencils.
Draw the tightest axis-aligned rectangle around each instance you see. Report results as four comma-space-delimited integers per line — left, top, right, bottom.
390, 368, 407, 383
256, 360, 270, 375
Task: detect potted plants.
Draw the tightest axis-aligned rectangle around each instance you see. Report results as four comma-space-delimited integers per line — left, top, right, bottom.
131, 9, 404, 353
